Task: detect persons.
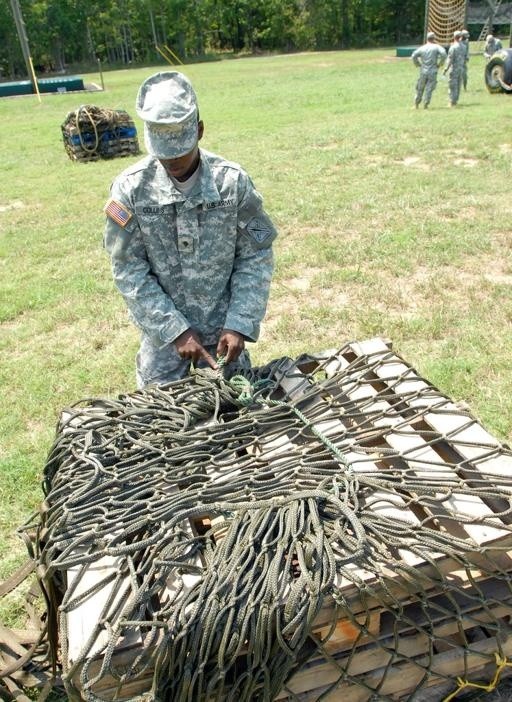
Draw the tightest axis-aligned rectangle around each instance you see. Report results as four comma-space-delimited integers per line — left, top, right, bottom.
484, 35, 502, 60
102, 70, 278, 389
411, 32, 447, 109
459, 30, 469, 92
442, 31, 466, 107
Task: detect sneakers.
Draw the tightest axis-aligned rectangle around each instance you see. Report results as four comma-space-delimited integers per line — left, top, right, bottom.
413, 103, 457, 109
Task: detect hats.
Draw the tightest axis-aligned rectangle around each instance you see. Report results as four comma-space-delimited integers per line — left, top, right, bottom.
427, 32, 434, 39
487, 35, 494, 43
454, 30, 469, 38
137, 71, 199, 159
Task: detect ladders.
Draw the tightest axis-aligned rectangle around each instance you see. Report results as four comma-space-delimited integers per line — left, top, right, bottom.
475, 0, 502, 51
